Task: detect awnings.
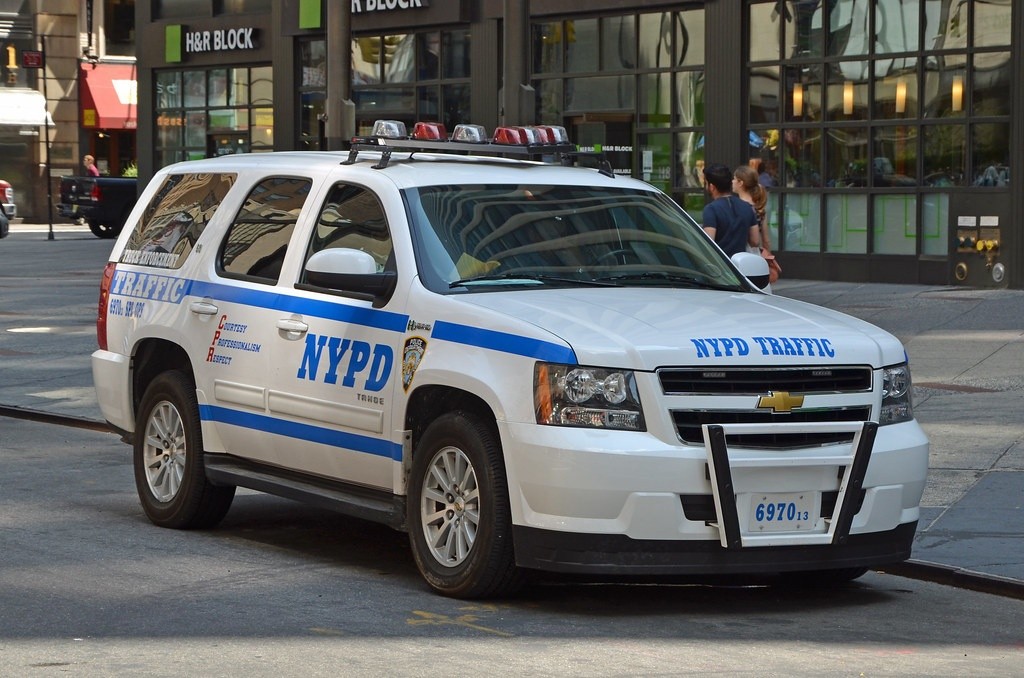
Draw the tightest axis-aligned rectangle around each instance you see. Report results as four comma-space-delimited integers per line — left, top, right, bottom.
0, 88, 55, 127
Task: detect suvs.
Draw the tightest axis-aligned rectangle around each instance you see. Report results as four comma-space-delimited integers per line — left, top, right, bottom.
90, 119, 929, 603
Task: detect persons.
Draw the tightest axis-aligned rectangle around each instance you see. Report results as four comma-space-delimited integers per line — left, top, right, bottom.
74, 155, 99, 226
982, 161, 1008, 187
701, 163, 781, 294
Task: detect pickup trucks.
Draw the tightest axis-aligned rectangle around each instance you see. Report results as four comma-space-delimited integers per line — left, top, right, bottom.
60, 173, 137, 240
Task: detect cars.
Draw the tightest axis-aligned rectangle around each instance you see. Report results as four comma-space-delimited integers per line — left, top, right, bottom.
844, 155, 1010, 186
